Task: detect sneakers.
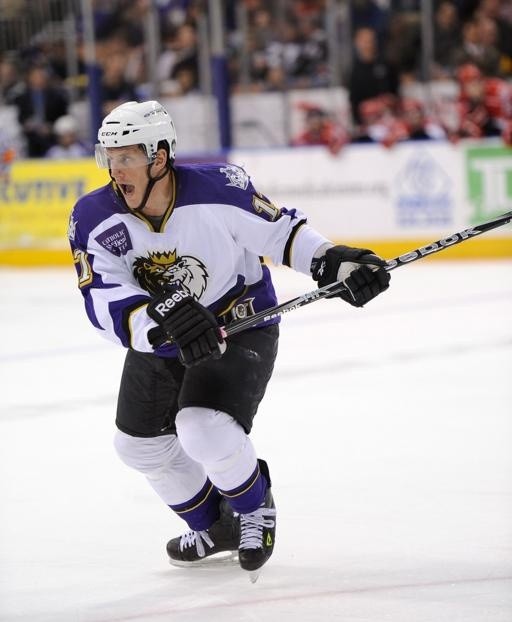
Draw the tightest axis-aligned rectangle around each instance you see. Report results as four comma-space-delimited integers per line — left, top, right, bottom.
239, 458, 277, 571
167, 495, 240, 562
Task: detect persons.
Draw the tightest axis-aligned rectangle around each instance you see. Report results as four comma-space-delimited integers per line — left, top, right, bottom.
64, 99, 393, 570
1, 0, 511, 167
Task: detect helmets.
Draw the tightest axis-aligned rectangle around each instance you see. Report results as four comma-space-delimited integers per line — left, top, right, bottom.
96, 100, 176, 168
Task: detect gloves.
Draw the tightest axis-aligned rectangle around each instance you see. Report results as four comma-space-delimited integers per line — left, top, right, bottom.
146, 287, 225, 365
313, 245, 391, 306
